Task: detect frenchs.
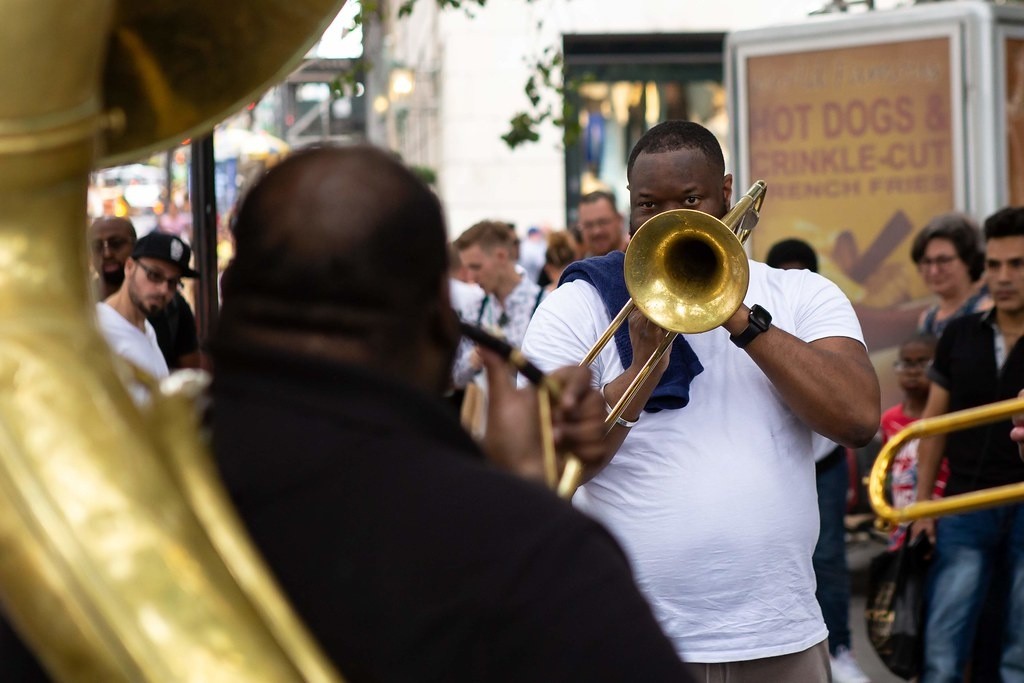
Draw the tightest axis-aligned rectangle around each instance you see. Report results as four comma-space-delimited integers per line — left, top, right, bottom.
1, 0, 349, 677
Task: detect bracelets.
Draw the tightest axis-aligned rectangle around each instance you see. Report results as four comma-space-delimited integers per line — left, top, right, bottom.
599, 383, 639, 428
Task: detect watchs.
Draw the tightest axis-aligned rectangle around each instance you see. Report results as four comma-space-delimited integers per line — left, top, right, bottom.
730, 304, 772, 348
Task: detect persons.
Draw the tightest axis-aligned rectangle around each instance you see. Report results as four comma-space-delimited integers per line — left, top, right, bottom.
764, 203, 1024, 682
201, 139, 693, 682
95, 231, 200, 407
521, 119, 882, 683
445, 189, 631, 484
88, 214, 200, 374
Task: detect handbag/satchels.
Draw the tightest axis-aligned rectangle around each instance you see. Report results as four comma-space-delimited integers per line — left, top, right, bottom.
861, 518, 932, 682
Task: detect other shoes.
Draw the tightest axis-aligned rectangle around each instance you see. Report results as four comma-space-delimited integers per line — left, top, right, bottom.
828, 652, 870, 683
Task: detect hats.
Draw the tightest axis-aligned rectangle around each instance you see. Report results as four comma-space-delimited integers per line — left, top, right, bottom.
132, 232, 198, 278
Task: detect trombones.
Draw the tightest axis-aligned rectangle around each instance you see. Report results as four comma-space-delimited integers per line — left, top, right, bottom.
576, 178, 770, 440
866, 395, 1023, 523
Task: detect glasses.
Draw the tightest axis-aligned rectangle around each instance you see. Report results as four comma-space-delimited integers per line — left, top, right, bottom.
89, 236, 135, 255
133, 257, 184, 291
917, 254, 959, 271
893, 357, 934, 374
